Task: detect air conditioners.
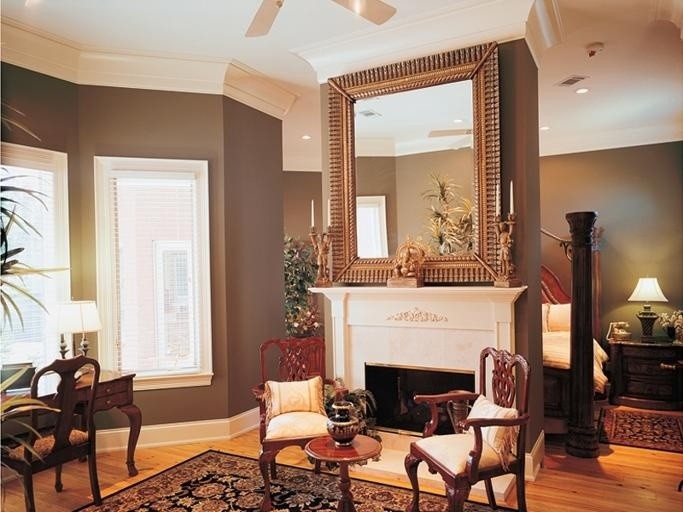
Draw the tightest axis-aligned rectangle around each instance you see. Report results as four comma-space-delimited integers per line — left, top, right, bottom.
320, 387, 380, 437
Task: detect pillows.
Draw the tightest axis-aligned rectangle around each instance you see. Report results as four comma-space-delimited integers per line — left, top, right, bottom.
540, 299, 571, 333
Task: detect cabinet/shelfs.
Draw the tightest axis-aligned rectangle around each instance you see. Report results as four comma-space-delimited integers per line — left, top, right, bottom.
606, 339, 682, 409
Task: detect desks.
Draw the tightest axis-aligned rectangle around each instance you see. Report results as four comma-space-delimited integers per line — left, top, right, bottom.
1, 361, 142, 481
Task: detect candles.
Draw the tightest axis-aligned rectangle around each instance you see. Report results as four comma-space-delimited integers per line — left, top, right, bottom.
327, 198, 333, 229
310, 200, 316, 229
493, 184, 500, 215
508, 179, 516, 215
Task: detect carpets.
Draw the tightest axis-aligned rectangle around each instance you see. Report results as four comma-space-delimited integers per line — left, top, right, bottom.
72, 445, 532, 512
595, 408, 682, 454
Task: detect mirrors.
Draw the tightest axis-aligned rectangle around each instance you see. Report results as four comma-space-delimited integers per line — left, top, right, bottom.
329, 43, 500, 282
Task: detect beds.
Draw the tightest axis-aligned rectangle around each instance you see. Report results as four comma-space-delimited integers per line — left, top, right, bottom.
541, 259, 608, 419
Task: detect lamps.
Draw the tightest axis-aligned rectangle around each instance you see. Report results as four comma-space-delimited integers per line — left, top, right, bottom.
64, 297, 104, 357
624, 276, 672, 344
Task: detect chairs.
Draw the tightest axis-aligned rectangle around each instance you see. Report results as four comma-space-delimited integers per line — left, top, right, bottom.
1, 356, 101, 512
249, 336, 344, 506
405, 345, 531, 508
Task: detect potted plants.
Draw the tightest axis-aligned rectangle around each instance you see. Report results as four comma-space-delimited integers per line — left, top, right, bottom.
282, 235, 322, 337
656, 307, 683, 341
